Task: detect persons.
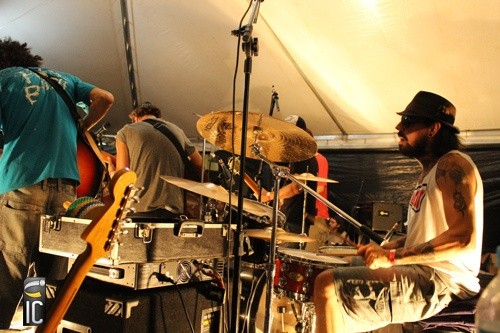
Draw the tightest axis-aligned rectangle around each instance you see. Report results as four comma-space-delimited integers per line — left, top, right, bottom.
253, 116, 353, 248
108, 101, 203, 222
0, 39, 115, 330
314, 91, 483, 333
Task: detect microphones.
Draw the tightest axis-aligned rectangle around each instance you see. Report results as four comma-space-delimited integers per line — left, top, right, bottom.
95, 122, 111, 136
215, 155, 235, 187
384, 222, 400, 242
272, 86, 280, 111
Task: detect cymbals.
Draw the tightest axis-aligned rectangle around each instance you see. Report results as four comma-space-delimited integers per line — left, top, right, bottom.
196, 110, 319, 162
281, 172, 339, 184
243, 226, 321, 246
158, 174, 266, 213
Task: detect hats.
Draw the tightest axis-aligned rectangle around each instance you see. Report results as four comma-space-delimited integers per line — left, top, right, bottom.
284, 115, 306, 130
397, 90, 461, 134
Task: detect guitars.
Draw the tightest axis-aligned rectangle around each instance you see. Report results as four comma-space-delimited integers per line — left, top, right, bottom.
34, 166, 145, 333
98, 148, 210, 205
74, 138, 104, 199
227, 155, 288, 213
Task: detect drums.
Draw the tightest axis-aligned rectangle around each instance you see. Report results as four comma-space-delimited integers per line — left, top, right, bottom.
273, 248, 352, 305
220, 198, 288, 265
319, 245, 358, 257
224, 260, 315, 333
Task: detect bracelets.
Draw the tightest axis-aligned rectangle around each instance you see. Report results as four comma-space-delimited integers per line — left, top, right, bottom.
390, 249, 396, 268
267, 192, 272, 202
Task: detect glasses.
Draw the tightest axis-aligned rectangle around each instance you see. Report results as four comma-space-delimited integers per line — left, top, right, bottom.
400, 115, 422, 128
